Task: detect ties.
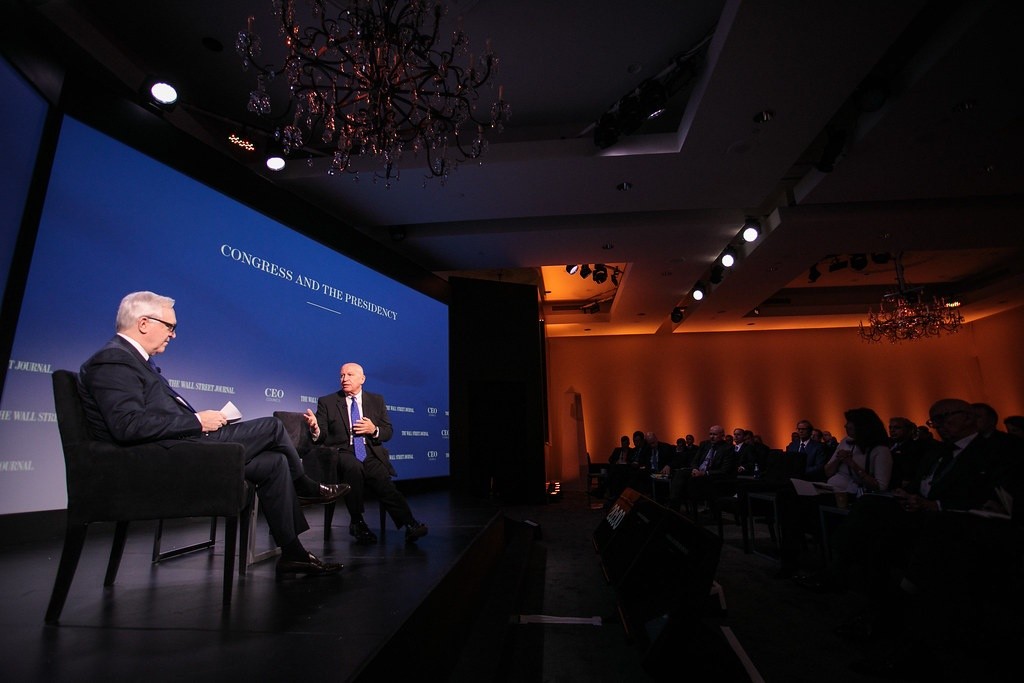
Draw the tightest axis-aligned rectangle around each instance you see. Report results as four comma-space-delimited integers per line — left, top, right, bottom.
149, 356, 196, 414
350, 396, 367, 462
701, 445, 715, 471
653, 448, 656, 469
800, 443, 806, 453
928, 443, 961, 503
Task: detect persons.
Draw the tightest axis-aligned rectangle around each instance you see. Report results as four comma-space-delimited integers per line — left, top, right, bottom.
77, 291, 351, 580
774, 408, 893, 580
609, 399, 1024, 680
304, 363, 428, 545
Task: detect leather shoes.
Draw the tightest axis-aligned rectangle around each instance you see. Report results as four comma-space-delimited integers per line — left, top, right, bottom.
349, 520, 377, 543
405, 523, 428, 539
297, 483, 351, 508
276, 551, 344, 574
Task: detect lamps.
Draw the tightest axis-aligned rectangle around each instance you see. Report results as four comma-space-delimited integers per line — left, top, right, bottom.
235, 1, 511, 189
671, 217, 758, 323
856, 251, 965, 345
594, 57, 698, 149
809, 252, 891, 283
566, 264, 619, 286
145, 74, 180, 113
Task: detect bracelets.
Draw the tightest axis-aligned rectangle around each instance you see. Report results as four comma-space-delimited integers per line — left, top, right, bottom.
707, 471, 709, 477
855, 467, 864, 476
371, 426, 377, 437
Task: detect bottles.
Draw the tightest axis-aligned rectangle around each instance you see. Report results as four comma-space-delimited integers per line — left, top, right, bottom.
755, 464, 760, 477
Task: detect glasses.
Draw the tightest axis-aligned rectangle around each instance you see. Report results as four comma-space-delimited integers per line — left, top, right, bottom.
795, 428, 809, 432
926, 410, 967, 428
137, 316, 176, 332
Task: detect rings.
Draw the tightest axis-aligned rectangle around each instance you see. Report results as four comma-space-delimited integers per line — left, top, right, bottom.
906, 509, 909, 512
906, 505, 909, 508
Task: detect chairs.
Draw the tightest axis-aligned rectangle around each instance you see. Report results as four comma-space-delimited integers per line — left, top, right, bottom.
587, 449, 1023, 640
45, 370, 257, 622
274, 411, 386, 541
